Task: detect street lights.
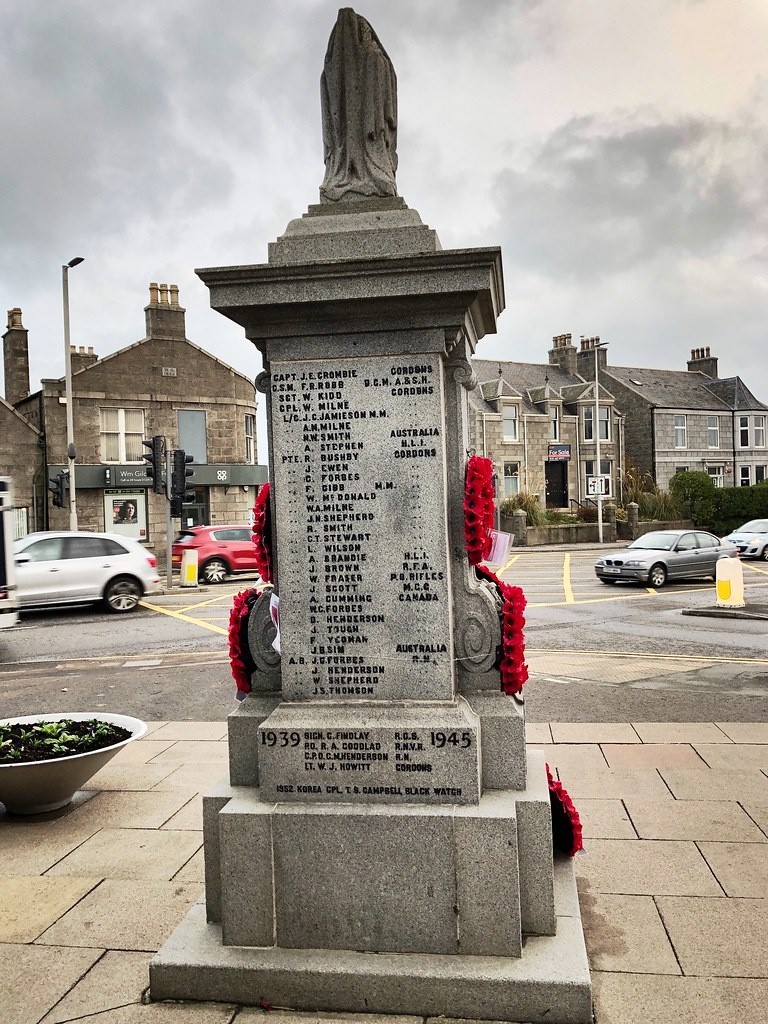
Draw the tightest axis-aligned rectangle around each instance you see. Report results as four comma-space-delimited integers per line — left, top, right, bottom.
61, 257, 85, 531
593, 342, 610, 544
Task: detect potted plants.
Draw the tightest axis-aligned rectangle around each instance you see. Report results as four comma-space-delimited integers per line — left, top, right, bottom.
0, 712, 147, 815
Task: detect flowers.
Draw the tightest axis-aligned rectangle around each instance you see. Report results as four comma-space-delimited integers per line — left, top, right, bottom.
463, 456, 496, 568
251, 482, 271, 582
227, 588, 262, 692
546, 763, 583, 857
474, 565, 530, 694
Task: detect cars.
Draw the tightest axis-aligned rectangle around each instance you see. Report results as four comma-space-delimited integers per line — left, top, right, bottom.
594, 530, 740, 588
721, 518, 768, 562
171, 525, 259, 584
13, 531, 162, 613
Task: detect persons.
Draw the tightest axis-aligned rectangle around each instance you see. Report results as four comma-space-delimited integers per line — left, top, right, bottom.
114, 501, 138, 524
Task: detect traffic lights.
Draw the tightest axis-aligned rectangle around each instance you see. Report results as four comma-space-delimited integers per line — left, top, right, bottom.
139, 436, 163, 495
49, 474, 68, 508
171, 449, 195, 516
